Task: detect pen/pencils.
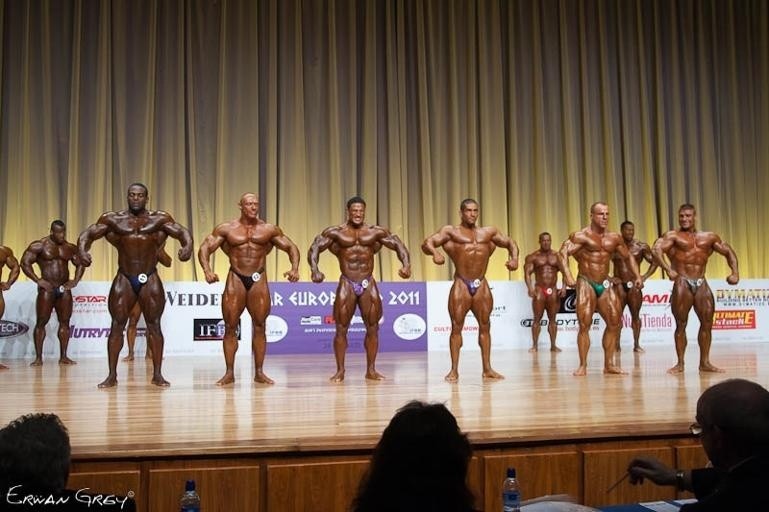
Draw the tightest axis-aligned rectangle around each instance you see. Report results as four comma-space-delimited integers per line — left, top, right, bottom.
605, 472, 632, 495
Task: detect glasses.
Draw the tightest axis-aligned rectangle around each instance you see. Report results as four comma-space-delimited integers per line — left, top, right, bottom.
689, 422, 713, 438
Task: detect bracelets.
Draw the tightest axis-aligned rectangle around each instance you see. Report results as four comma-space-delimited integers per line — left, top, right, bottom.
676, 471, 685, 492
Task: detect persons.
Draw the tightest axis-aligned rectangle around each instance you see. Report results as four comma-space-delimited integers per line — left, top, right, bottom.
21, 220, 86, 366
123, 239, 173, 361
626, 379, 769, 510
0, 245, 19, 369
422, 199, 520, 379
199, 193, 300, 386
608, 222, 658, 351
0, 415, 71, 510
557, 203, 643, 375
524, 232, 564, 352
308, 197, 411, 382
349, 399, 474, 512
650, 204, 739, 373
77, 184, 193, 389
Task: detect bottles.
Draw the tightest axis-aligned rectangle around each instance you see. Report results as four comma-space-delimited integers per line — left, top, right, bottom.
177, 479, 201, 512
502, 467, 520, 512
